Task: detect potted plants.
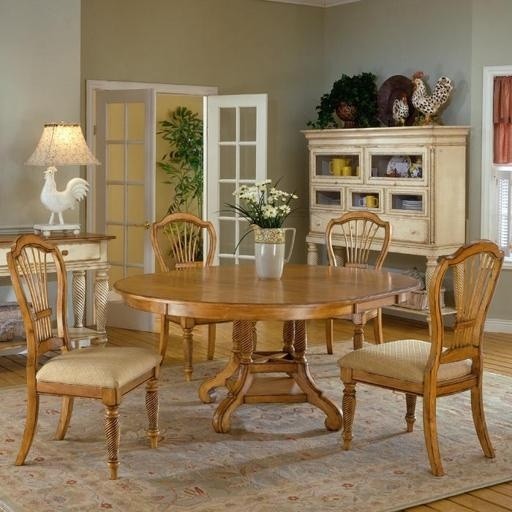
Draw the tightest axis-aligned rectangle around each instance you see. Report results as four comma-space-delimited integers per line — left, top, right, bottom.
308, 70, 382, 128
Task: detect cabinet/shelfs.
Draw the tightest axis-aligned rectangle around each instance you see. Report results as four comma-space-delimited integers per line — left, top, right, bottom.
303, 126, 469, 320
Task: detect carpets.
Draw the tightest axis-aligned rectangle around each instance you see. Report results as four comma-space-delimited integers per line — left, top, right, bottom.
1, 338, 512, 511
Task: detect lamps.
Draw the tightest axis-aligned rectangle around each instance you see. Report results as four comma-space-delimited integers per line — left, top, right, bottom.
25, 123, 103, 241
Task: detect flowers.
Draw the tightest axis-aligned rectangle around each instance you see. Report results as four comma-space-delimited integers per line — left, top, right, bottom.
215, 179, 299, 250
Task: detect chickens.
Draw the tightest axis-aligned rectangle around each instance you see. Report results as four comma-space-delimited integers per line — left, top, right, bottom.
410, 70, 453, 124
40, 166, 90, 227
391, 93, 409, 127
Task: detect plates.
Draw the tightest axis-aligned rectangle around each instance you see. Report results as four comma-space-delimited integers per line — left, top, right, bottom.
387, 156, 411, 177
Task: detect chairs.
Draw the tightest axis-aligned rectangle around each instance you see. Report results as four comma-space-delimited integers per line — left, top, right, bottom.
4, 231, 162, 481
336, 238, 504, 480
324, 212, 392, 355
151, 211, 233, 386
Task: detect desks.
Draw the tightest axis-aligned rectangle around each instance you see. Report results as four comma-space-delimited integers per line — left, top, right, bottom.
0, 232, 117, 348
112, 260, 424, 435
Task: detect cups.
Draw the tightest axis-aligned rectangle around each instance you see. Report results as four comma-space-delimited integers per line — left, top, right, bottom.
328, 158, 352, 176
359, 196, 378, 208
